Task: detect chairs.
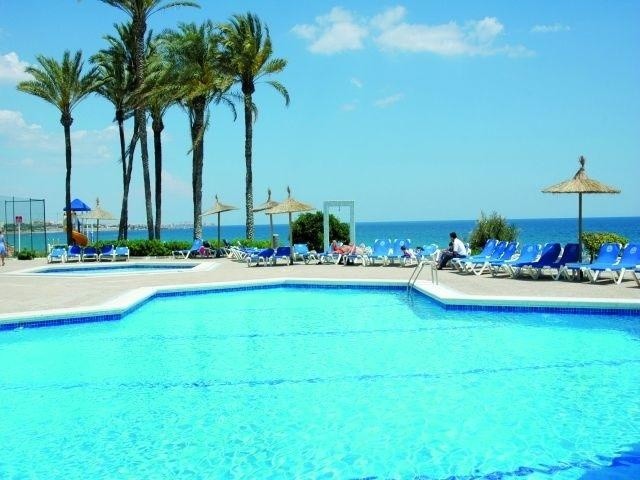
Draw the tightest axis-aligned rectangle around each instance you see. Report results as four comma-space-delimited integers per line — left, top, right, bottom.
172, 239, 203, 260
47, 244, 130, 262
445, 239, 640, 288
293, 239, 438, 268
208, 246, 291, 268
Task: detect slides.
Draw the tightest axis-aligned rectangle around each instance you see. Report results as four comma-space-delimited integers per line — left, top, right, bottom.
64, 227, 88, 246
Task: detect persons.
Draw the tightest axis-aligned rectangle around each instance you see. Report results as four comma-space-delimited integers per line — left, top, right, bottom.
0, 231, 7, 266
432, 232, 466, 270
400, 245, 412, 268
